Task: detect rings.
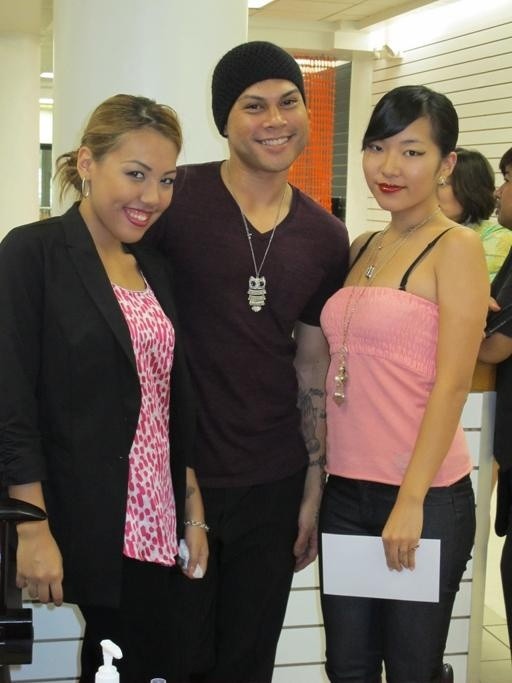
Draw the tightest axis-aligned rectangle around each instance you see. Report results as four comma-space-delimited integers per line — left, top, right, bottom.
409, 542, 421, 555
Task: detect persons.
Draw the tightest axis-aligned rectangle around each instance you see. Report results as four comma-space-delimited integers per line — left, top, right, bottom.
0, 92, 209, 681
149, 39, 355, 681
314, 82, 489, 681
476, 147, 512, 658
435, 143, 497, 233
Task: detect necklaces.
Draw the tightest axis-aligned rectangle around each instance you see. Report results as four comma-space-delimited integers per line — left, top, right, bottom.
224, 155, 292, 321
329, 205, 442, 408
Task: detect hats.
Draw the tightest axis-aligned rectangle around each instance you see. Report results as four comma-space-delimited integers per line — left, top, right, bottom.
211, 40, 307, 138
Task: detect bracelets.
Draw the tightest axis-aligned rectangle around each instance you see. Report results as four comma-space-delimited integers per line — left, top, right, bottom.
181, 514, 212, 535
